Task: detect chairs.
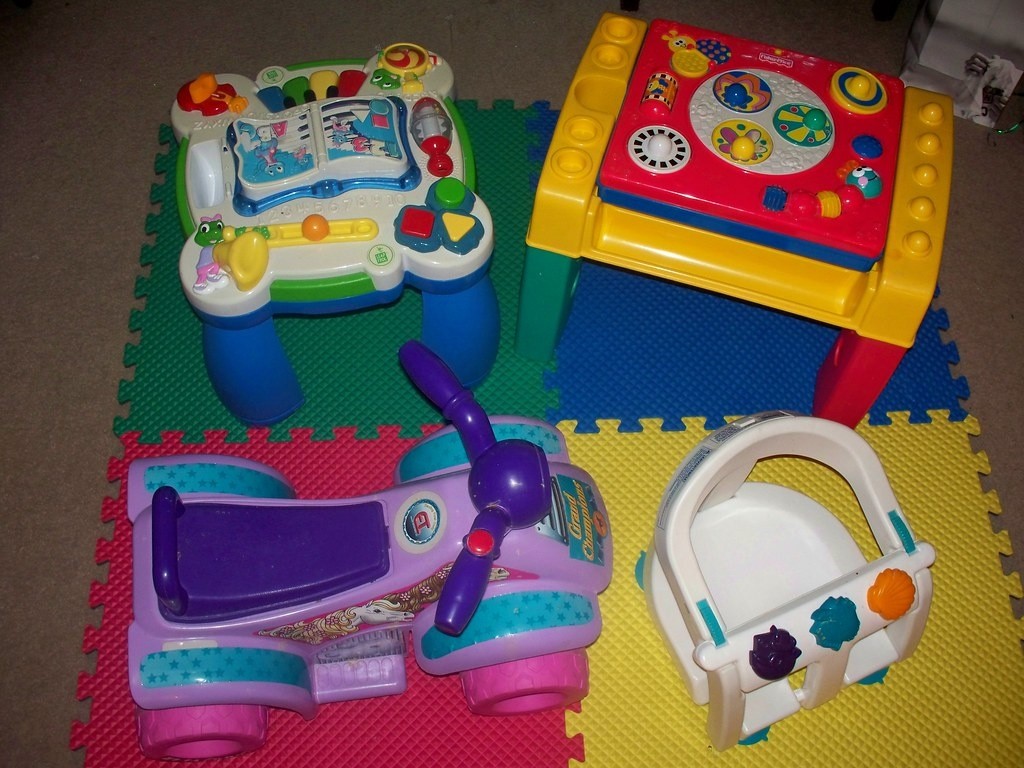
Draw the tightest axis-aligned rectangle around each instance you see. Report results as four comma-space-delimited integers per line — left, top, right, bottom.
644, 415, 936, 750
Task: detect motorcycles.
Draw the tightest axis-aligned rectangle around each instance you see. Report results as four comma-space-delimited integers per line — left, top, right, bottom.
127, 339, 614, 760
